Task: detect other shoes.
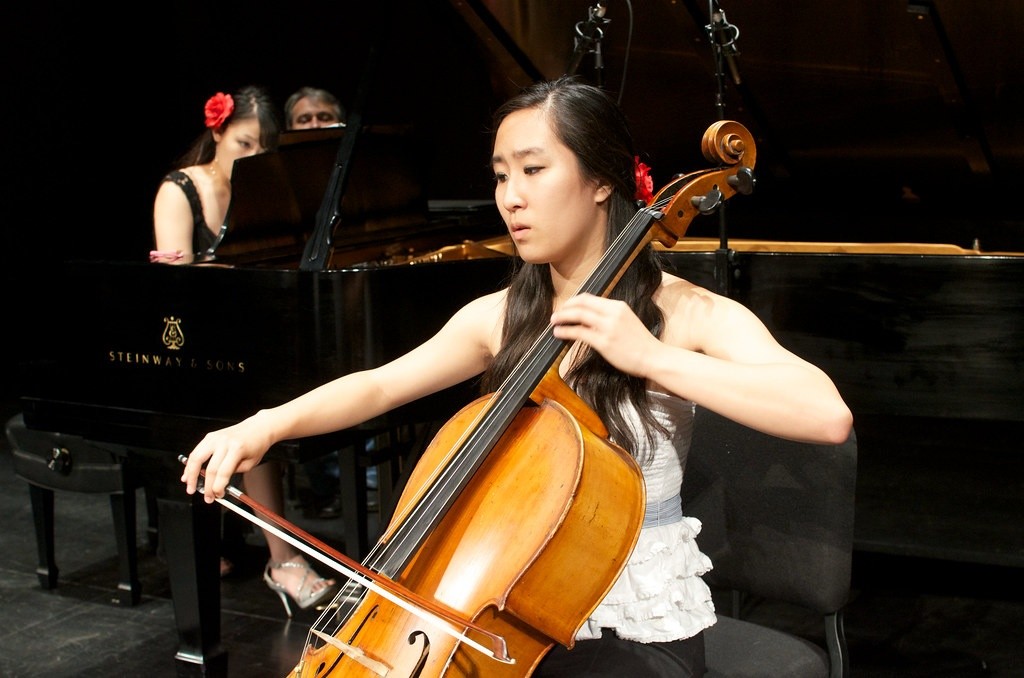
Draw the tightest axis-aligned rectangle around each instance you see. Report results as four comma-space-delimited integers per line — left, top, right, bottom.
295, 484, 378, 520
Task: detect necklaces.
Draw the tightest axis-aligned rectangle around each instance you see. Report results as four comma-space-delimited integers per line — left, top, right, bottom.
210, 164, 217, 177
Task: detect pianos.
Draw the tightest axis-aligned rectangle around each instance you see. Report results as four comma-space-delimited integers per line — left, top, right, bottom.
16, 0, 1024, 678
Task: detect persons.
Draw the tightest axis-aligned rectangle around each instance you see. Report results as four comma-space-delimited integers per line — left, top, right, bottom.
178, 75, 854, 678
283, 87, 344, 132
152, 81, 336, 615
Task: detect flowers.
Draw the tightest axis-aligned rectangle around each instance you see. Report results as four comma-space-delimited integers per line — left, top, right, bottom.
203, 92, 235, 129
634, 156, 653, 204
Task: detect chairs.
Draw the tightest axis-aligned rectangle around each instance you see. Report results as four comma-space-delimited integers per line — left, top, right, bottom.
681, 405, 860, 678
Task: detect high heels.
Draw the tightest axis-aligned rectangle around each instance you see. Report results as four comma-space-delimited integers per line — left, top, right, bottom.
220, 525, 251, 581
264, 559, 339, 618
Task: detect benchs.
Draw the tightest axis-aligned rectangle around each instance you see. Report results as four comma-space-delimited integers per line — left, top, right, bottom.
849, 410, 1023, 573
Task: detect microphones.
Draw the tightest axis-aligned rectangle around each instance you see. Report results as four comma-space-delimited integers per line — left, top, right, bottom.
712, 0, 740, 84
567, 1, 607, 78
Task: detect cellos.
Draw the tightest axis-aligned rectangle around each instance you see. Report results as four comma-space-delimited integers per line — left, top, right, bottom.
288, 118, 757, 678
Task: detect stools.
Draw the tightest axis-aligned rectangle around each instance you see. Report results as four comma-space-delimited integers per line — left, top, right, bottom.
4, 413, 159, 609
